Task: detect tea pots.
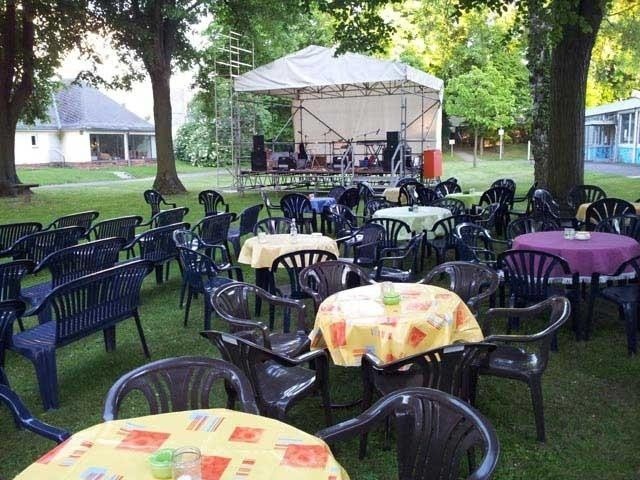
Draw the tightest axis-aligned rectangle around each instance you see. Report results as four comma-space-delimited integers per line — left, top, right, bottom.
576, 231, 592, 241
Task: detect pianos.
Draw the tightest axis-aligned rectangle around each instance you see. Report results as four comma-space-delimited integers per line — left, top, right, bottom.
356, 140, 387, 145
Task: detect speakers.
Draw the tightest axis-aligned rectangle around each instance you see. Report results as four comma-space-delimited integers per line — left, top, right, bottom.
383, 147, 401, 173
251, 152, 267, 171
387, 131, 400, 149
252, 135, 264, 153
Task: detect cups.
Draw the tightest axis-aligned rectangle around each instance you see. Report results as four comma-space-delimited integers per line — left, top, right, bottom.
309, 193, 314, 200
259, 232, 266, 243
170, 448, 201, 480
564, 228, 575, 241
381, 280, 394, 299
469, 187, 475, 196
413, 205, 418, 213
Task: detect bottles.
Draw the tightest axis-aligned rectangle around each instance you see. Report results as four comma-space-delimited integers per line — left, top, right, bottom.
462, 189, 469, 194
148, 452, 177, 478
409, 207, 414, 212
290, 218, 298, 243
383, 290, 400, 305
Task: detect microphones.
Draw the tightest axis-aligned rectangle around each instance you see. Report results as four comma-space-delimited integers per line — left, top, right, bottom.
376, 129, 381, 135
325, 128, 333, 134
297, 131, 305, 135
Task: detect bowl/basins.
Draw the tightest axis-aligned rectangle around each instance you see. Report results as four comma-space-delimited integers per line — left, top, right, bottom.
312, 233, 323, 239
